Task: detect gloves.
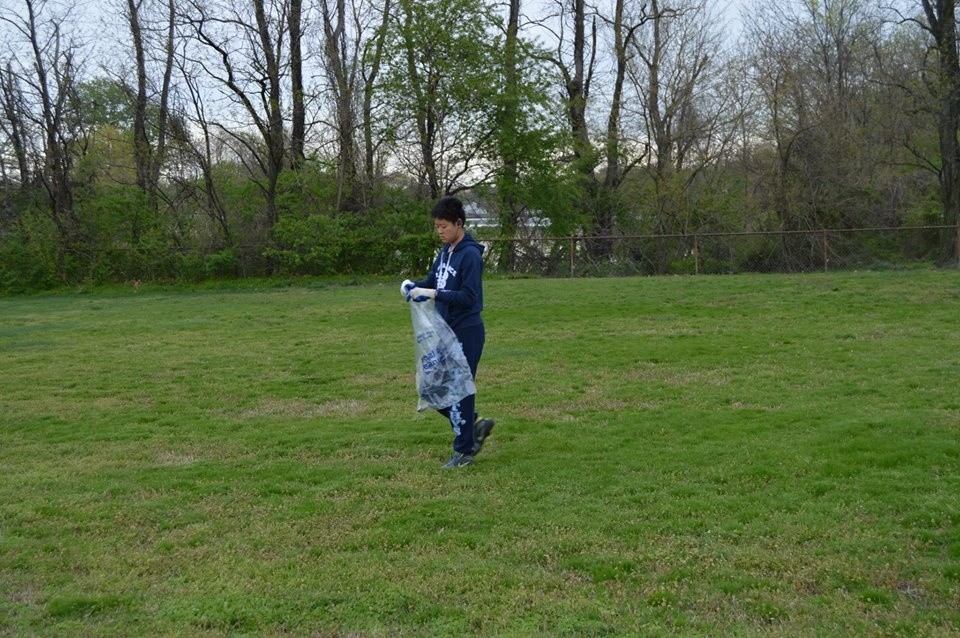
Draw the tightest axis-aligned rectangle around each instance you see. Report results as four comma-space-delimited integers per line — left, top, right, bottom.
406, 287, 436, 303
400, 279, 415, 297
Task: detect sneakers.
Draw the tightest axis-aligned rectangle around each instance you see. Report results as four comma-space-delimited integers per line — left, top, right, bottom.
440, 451, 474, 469
471, 417, 494, 456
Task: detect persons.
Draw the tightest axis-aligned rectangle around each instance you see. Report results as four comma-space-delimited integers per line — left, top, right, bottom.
400, 197, 495, 471
